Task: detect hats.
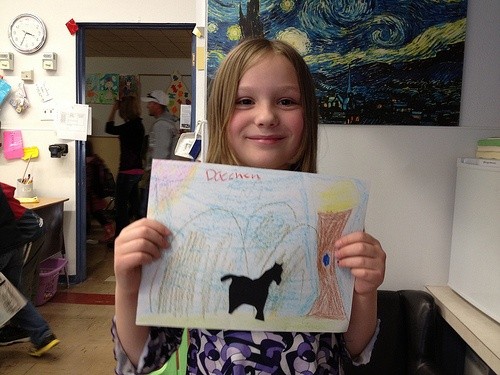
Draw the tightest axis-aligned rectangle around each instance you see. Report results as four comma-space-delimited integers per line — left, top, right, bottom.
141, 89, 169, 106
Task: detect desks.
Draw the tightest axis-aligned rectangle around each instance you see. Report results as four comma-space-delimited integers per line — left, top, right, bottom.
17, 196, 70, 288
428, 281, 500, 375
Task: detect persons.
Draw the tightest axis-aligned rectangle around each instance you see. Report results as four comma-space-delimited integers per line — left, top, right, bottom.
0, 183, 64, 357
111, 38, 387, 375
85, 91, 171, 248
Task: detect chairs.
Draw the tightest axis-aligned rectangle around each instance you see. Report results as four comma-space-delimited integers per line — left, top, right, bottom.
347, 290, 441, 375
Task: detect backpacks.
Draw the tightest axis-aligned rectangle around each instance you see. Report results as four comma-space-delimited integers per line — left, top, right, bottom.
149, 117, 181, 160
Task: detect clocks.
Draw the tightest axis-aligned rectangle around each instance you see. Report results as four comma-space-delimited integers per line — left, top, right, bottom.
9, 13, 47, 53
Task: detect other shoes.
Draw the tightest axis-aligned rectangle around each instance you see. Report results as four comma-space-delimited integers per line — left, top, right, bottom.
28, 333, 60, 357
0, 326, 30, 345
